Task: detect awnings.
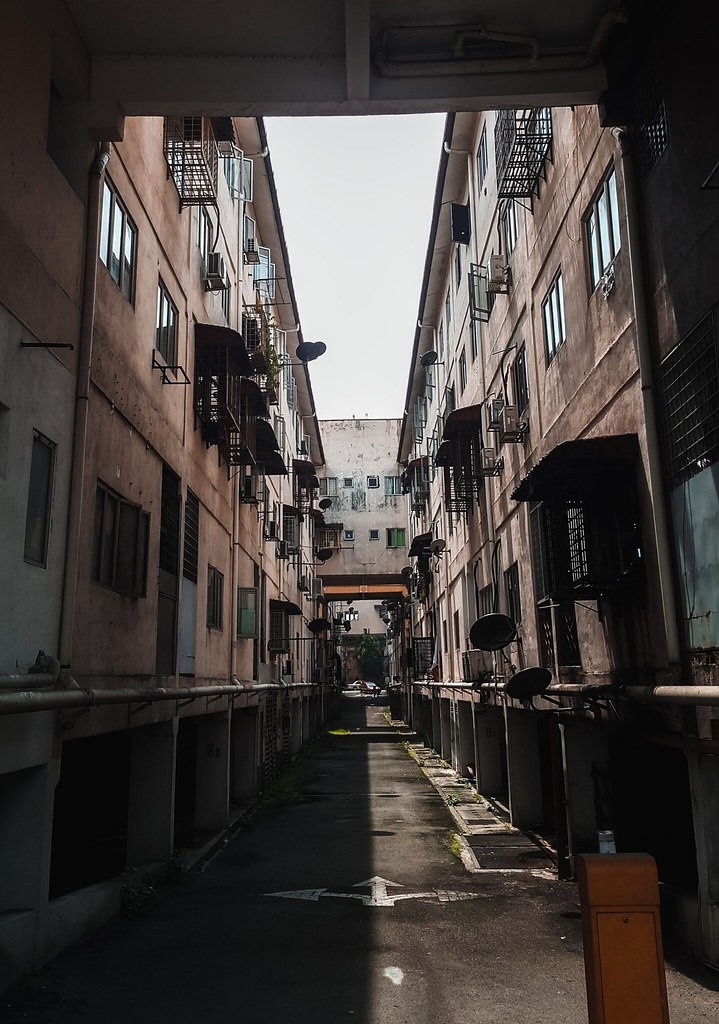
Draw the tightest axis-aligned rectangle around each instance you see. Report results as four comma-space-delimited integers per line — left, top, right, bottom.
403, 457, 428, 486
509, 433, 638, 501
271, 598, 303, 616
291, 458, 320, 490
408, 532, 433, 557
196, 322, 288, 475
434, 403, 483, 467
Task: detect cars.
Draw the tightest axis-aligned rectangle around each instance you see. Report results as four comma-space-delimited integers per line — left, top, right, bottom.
358, 682, 382, 695
347, 680, 363, 691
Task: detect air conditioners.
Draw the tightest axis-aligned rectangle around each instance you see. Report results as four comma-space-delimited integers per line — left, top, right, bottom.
285, 659, 295, 674
299, 575, 309, 589
314, 545, 319, 554
480, 447, 495, 476
246, 236, 260, 261
460, 649, 486, 683
499, 406, 520, 440
280, 540, 289, 556
301, 440, 307, 453
244, 474, 258, 501
487, 398, 504, 427
207, 252, 227, 288
267, 521, 279, 540
485, 255, 505, 291
